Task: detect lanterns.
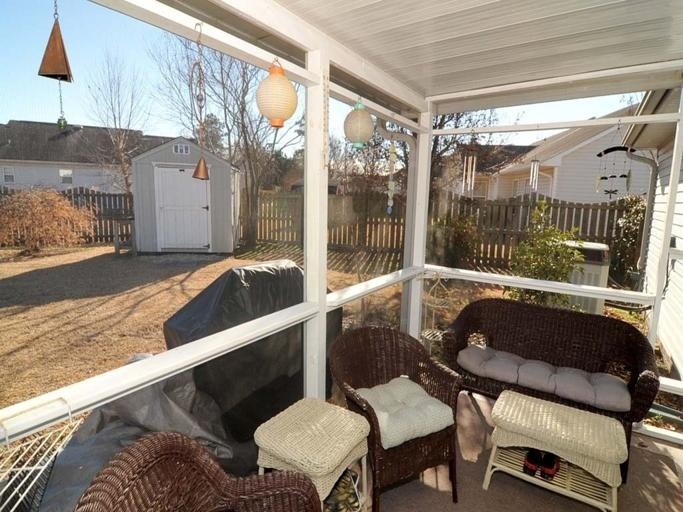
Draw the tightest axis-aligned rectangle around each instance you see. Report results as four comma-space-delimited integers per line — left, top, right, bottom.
251, 65, 298, 128
342, 101, 375, 150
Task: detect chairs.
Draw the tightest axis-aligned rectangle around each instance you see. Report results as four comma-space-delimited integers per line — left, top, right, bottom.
72, 428, 325, 511
323, 322, 463, 511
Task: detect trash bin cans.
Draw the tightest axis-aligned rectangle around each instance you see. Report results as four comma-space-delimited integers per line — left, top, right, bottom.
563, 240, 610, 315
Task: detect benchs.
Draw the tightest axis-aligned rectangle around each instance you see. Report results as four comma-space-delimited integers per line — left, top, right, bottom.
440, 295, 661, 484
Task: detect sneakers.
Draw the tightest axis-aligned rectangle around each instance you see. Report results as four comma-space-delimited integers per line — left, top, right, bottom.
541, 453, 561, 481
524, 451, 542, 477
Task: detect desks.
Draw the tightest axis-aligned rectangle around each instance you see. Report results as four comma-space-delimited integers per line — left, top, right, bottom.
250, 393, 374, 510
482, 382, 628, 510
